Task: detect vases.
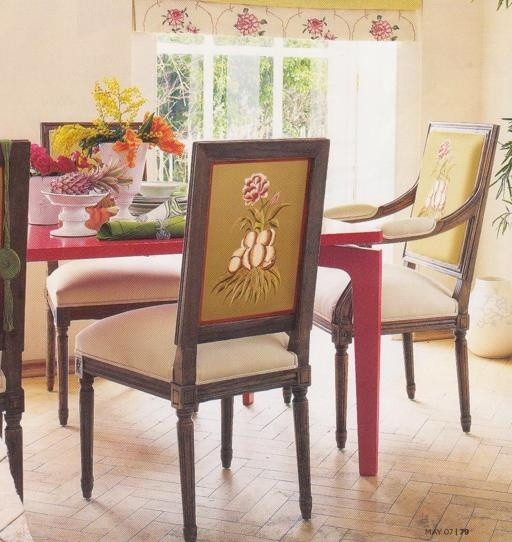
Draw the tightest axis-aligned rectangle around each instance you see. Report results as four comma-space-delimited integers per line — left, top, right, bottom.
466, 276, 512, 359
97, 143, 149, 223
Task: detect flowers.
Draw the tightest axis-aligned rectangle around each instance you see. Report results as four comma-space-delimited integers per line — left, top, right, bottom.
300, 16, 338, 43
211, 171, 289, 304
30, 74, 184, 175
160, 7, 198, 35
232, 7, 268, 37
367, 16, 400, 43
417, 139, 454, 217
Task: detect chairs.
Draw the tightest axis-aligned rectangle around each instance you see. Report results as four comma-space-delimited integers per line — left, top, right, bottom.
71, 137, 330, 541
0, 137, 33, 504
311, 120, 501, 449
39, 121, 184, 425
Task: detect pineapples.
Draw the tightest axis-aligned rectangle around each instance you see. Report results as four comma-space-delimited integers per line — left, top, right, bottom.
50, 156, 133, 196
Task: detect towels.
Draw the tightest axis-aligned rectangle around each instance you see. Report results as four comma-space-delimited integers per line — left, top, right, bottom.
96, 216, 188, 241
